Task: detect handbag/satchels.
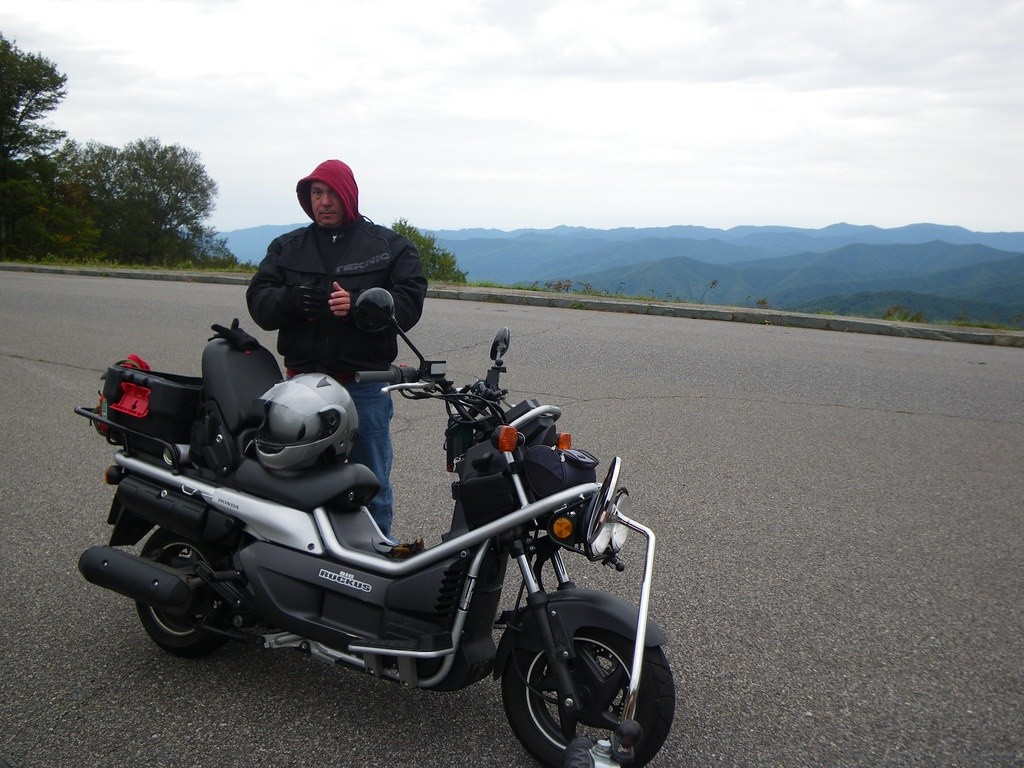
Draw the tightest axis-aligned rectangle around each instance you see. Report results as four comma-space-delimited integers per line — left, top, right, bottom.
523, 444, 600, 501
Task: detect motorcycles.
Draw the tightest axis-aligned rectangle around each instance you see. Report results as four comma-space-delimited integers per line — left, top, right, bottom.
73, 289, 677, 768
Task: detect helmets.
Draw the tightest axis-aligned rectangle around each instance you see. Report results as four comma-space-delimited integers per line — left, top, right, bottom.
254, 373, 358, 475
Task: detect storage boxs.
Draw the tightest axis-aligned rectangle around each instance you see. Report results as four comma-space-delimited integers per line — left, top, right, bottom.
102, 364, 208, 445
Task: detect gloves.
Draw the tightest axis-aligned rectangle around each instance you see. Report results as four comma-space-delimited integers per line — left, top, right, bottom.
207, 318, 258, 350
283, 278, 328, 323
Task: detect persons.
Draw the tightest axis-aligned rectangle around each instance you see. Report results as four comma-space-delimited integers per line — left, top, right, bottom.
247, 160, 428, 546
